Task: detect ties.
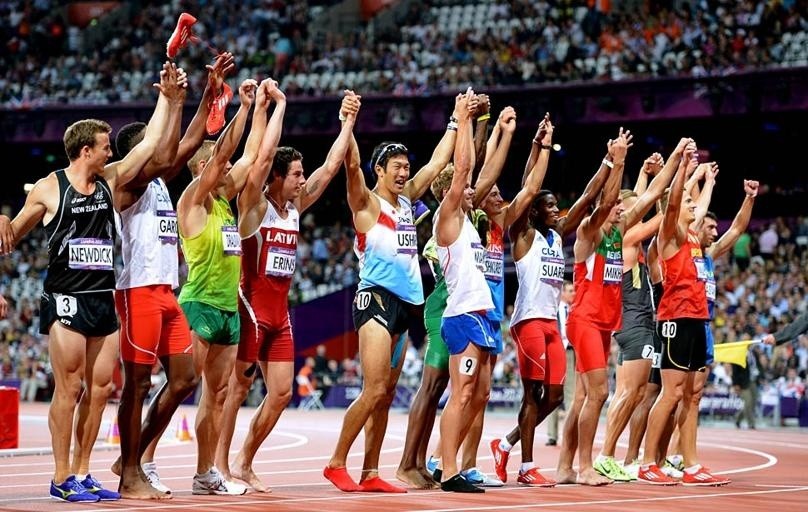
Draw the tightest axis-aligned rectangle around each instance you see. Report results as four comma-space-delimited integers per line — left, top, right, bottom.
564, 306, 567, 317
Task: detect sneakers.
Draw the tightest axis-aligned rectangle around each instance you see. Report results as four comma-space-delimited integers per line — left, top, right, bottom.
141, 461, 173, 493
636, 465, 681, 485
592, 455, 631, 482
49, 475, 101, 503
490, 438, 509, 483
459, 467, 505, 486
425, 455, 440, 475
192, 465, 247, 495
517, 467, 557, 487
621, 457, 640, 480
166, 12, 197, 60
667, 455, 685, 471
421, 238, 438, 263
81, 473, 121, 500
660, 459, 684, 478
206, 77, 234, 135
681, 467, 731, 486
471, 209, 489, 249
411, 200, 430, 227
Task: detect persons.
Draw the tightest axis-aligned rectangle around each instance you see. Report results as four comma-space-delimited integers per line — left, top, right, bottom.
12, 67, 188, 504
0, 295, 8, 321
212, 83, 363, 495
322, 86, 760, 494
0, 182, 806, 431
0, 213, 11, 256
111, 51, 235, 501
141, 77, 278, 495
1, 3, 808, 111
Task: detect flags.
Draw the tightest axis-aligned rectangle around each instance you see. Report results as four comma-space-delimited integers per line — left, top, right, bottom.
713, 341, 752, 369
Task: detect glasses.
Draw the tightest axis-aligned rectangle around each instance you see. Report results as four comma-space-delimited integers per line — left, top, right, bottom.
375, 143, 408, 165
